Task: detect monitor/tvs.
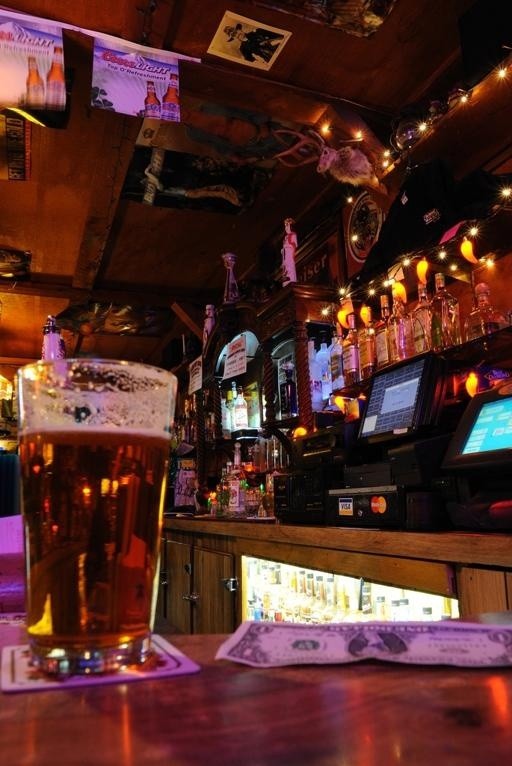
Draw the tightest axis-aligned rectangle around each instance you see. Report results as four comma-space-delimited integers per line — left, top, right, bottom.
355, 350, 439, 446
438, 388, 511, 469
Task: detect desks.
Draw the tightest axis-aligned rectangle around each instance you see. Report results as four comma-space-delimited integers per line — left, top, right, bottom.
0, 621, 510, 766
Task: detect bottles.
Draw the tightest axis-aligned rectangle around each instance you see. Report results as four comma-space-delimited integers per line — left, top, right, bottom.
468, 283, 504, 334
375, 297, 392, 369
389, 286, 412, 358
429, 271, 465, 348
341, 316, 359, 383
199, 389, 286, 516
360, 305, 377, 374
411, 281, 429, 352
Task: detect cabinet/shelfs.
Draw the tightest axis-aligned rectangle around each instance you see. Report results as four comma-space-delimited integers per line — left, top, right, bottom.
157, 531, 240, 635
327, 50, 511, 430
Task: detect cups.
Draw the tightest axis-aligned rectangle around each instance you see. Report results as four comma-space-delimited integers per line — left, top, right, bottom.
17, 362, 179, 684
